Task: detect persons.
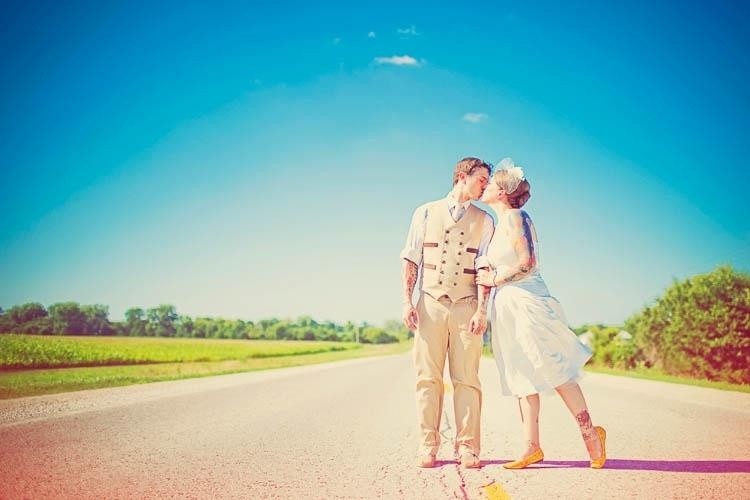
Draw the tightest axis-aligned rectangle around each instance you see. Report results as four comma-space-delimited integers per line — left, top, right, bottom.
474, 166, 609, 471
398, 153, 492, 470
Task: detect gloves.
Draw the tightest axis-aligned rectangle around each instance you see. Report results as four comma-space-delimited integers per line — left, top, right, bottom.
474, 268, 497, 287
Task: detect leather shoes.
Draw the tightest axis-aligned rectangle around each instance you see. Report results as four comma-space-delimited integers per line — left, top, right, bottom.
502, 447, 545, 469
590, 425, 607, 470
454, 447, 481, 467
415, 451, 437, 468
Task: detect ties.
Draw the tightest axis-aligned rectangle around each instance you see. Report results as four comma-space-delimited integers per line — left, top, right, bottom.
451, 204, 464, 223
